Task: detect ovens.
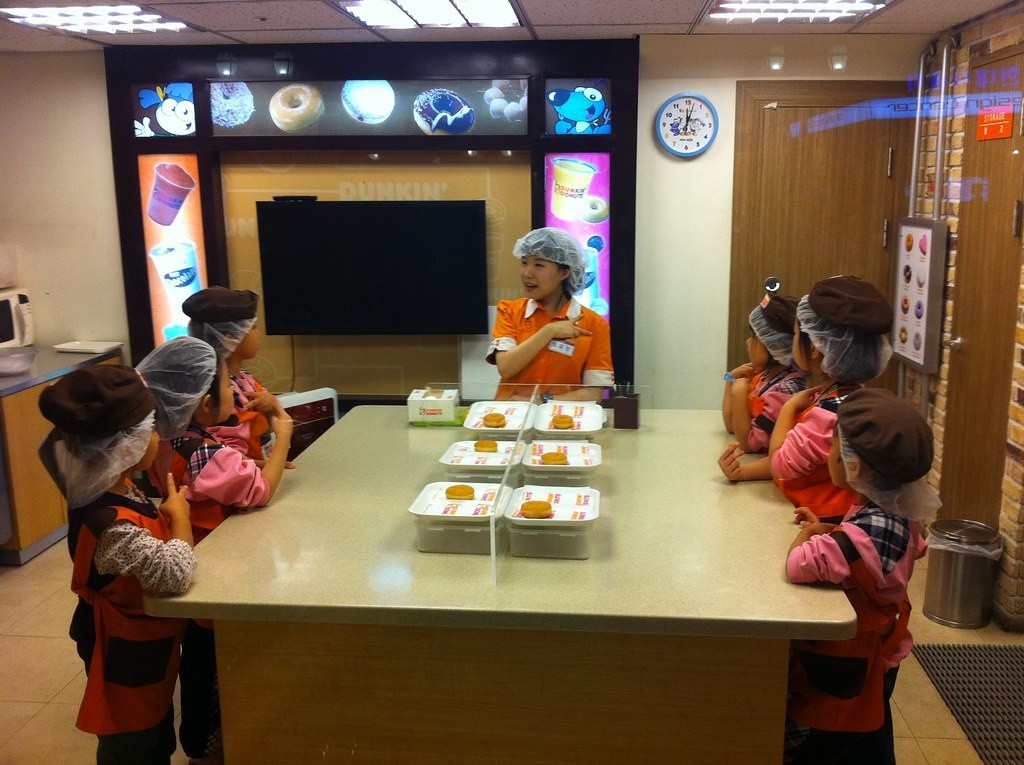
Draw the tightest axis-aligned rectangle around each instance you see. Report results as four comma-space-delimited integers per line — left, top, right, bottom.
0, 286, 35, 350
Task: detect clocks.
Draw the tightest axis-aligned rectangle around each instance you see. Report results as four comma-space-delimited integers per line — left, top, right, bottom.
655, 92, 718, 157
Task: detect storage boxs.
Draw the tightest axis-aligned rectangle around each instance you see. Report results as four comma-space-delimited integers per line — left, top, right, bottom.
407, 387, 607, 560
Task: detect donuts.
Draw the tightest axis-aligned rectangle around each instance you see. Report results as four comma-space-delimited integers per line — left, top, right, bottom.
209, 82, 254, 126
552, 414, 574, 429
483, 413, 506, 427
445, 485, 474, 500
269, 84, 324, 132
899, 234, 927, 350
341, 80, 395, 124
474, 440, 497, 452
521, 501, 552, 517
413, 88, 476, 135
542, 452, 567, 464
579, 196, 609, 223
483, 78, 528, 122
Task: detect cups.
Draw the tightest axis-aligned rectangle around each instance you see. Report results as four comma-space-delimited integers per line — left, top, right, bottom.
147, 163, 195, 225
149, 240, 203, 316
570, 247, 600, 310
551, 157, 597, 221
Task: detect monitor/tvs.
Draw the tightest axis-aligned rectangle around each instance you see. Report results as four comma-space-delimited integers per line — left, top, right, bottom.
256, 200, 488, 335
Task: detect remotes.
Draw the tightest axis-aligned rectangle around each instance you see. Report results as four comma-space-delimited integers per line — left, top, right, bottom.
273, 195, 317, 202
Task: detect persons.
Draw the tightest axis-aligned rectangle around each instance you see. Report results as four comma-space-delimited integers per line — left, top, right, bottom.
35, 364, 196, 765
723, 292, 804, 453
136, 335, 292, 765
786, 389, 934, 765
184, 286, 295, 469
720, 277, 891, 525
484, 226, 614, 405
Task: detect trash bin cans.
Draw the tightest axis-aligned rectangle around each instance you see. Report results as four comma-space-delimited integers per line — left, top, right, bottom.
921, 518, 1002, 630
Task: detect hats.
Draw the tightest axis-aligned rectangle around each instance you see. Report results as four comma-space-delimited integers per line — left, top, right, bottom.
836, 387, 935, 484
38, 364, 155, 438
760, 292, 805, 335
808, 274, 897, 334
182, 286, 262, 323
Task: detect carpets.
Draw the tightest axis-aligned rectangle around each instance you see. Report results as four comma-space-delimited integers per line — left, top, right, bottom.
910, 644, 1024, 765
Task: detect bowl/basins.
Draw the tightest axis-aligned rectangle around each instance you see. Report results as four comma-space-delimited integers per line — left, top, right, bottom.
0, 347, 40, 377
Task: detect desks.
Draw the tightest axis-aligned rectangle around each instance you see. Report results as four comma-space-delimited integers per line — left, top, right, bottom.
0, 342, 126, 566
145, 406, 858, 764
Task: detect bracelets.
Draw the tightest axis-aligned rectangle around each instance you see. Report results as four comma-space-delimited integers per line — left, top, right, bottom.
541, 392, 553, 403
724, 371, 737, 383
